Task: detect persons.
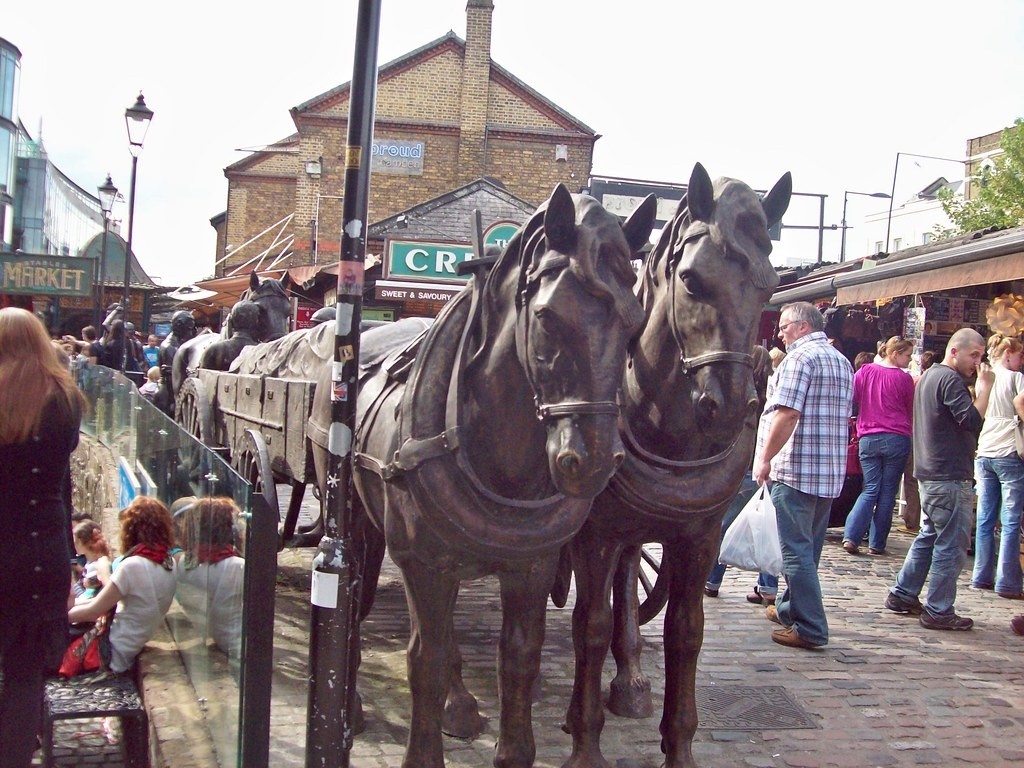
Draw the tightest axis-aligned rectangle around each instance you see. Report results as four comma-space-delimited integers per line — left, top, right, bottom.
310, 307, 338, 325
704, 302, 853, 649
201, 299, 261, 370
0, 307, 88, 768
161, 311, 195, 421
58, 321, 163, 399
843, 329, 1024, 636
170, 496, 245, 660
52, 495, 178, 685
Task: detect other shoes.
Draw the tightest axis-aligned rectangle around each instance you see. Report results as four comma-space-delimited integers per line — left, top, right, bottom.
974, 582, 995, 589
747, 591, 776, 605
843, 541, 859, 554
1010, 616, 1024, 634
868, 548, 886, 555
996, 584, 1023, 599
704, 586, 719, 597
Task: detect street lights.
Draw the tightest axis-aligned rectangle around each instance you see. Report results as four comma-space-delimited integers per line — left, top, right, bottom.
840, 191, 893, 264
117, 90, 155, 373
884, 152, 987, 253
95, 174, 120, 337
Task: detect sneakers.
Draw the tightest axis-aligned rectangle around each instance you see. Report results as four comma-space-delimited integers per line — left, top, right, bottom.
885, 591, 925, 615
772, 626, 819, 648
766, 604, 780, 623
920, 608, 973, 630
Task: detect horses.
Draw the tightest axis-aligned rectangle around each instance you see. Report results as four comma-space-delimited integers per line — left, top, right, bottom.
170, 269, 290, 411
309, 161, 793, 768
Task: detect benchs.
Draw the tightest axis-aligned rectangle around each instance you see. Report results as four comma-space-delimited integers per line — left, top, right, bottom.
38, 675, 149, 768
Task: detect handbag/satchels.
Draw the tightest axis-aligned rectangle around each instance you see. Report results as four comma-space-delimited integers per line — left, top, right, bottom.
846, 441, 863, 474
718, 481, 783, 577
1011, 371, 1024, 461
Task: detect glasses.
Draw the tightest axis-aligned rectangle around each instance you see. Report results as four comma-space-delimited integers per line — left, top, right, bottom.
779, 321, 801, 331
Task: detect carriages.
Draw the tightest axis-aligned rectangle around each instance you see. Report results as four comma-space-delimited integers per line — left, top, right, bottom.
180, 157, 795, 768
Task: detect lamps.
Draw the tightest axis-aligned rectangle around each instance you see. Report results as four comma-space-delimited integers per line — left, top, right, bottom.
555, 143, 568, 163
396, 213, 458, 242
305, 156, 323, 179
